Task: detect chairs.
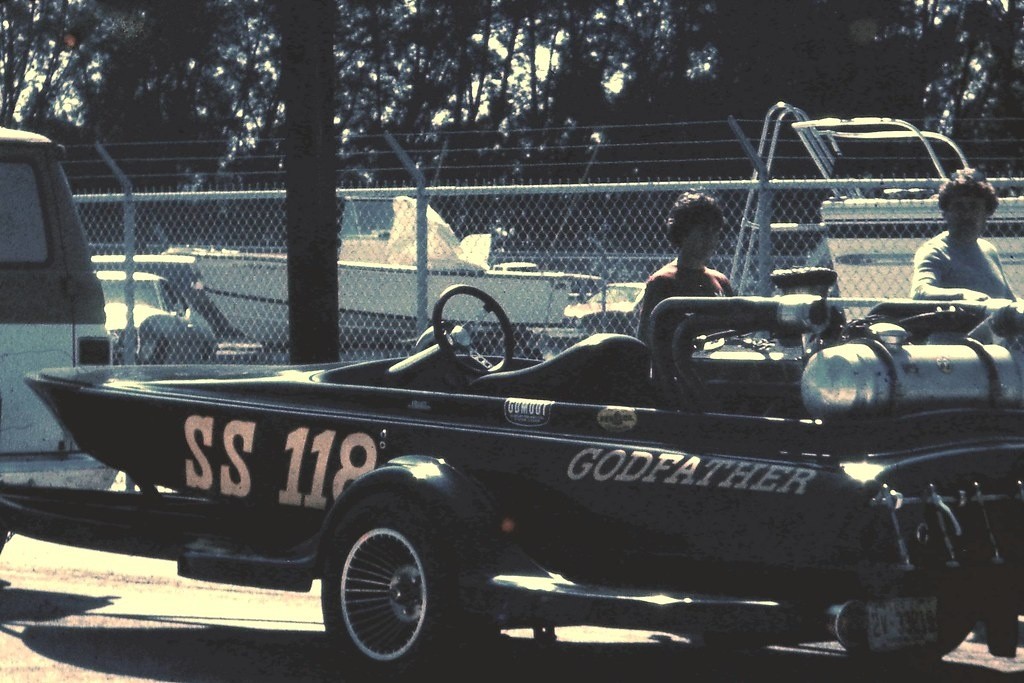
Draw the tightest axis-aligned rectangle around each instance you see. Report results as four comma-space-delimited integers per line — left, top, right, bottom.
473, 330, 644, 401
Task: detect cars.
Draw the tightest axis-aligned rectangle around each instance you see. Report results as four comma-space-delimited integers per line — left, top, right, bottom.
565, 283, 649, 323
0, 122, 121, 559
92, 271, 218, 363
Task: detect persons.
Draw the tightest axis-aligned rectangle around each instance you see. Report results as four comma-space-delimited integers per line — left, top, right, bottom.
912, 167, 1017, 301
638, 189, 744, 347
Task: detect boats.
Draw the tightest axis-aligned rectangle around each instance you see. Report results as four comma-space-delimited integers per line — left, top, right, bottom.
155, 242, 604, 351
25, 266, 1023, 673
820, 176, 1024, 325
